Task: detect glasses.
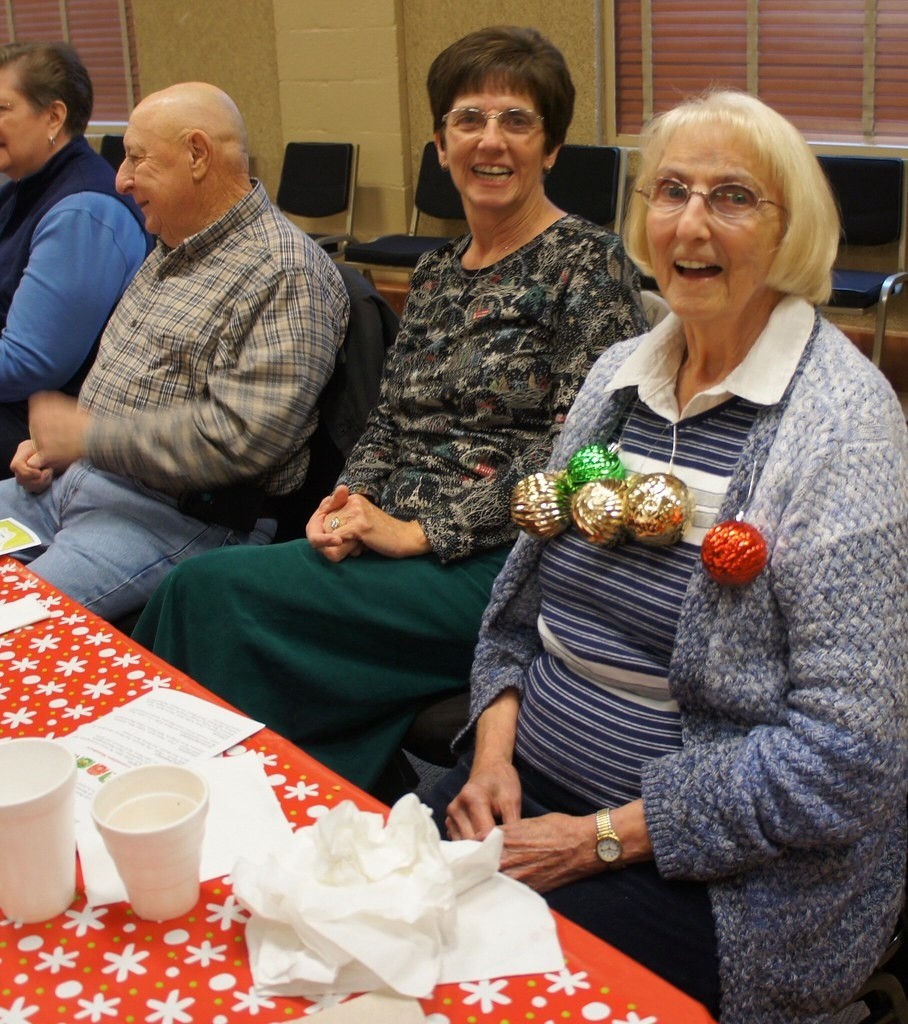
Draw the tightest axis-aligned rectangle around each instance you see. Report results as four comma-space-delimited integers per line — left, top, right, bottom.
633, 174, 790, 221
441, 107, 545, 134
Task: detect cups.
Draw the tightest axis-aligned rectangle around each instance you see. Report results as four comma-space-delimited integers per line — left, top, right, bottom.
90, 767, 210, 921
0, 738, 77, 923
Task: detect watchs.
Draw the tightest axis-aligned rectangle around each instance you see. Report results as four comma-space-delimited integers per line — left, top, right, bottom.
594, 807, 625, 869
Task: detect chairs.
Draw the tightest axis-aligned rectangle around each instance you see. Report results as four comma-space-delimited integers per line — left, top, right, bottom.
341, 142, 465, 286
276, 141, 360, 263
812, 154, 908, 371
543, 142, 626, 238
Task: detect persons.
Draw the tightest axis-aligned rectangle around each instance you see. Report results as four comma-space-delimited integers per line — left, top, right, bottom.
415, 90, 908, 1024
0, 38, 160, 483
0, 79, 352, 625
126, 23, 656, 804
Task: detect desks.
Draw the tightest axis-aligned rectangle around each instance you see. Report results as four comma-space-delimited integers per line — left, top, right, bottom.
1, 552, 711, 1024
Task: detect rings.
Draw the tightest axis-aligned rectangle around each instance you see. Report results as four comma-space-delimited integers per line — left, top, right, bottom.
330, 517, 339, 530
